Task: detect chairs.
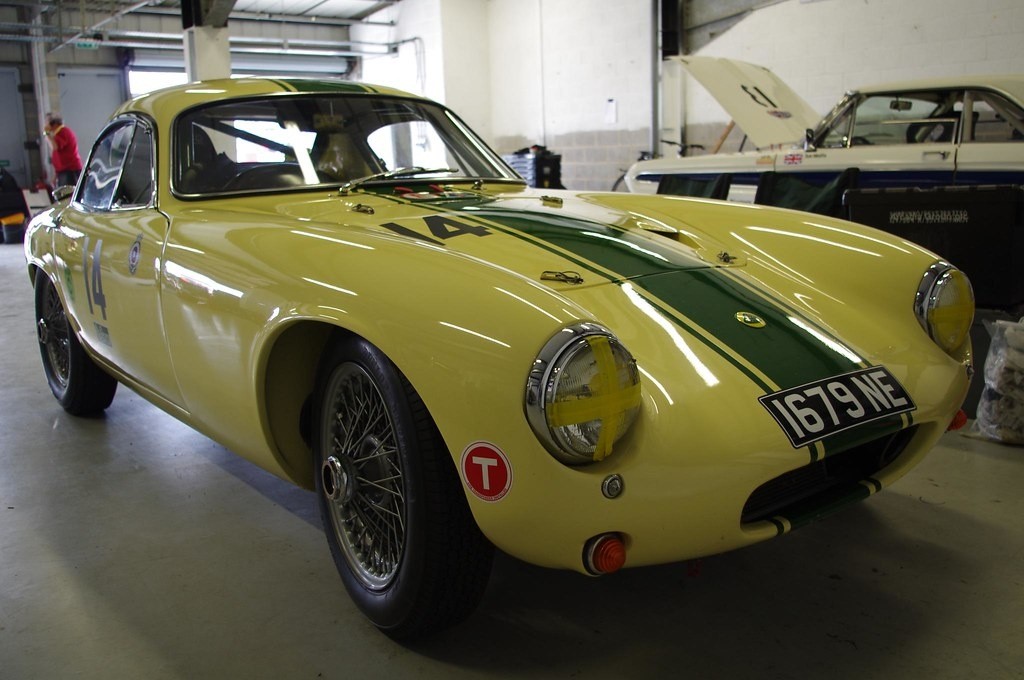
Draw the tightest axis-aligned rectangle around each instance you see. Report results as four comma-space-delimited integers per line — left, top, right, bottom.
122, 122, 220, 203
908, 111, 977, 143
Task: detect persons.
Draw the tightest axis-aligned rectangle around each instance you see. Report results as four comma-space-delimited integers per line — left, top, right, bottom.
43, 111, 82, 188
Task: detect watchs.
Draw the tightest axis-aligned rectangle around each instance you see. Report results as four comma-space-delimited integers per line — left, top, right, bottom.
43, 131, 50, 136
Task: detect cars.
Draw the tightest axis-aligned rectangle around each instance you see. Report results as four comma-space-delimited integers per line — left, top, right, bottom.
20, 70, 978, 645
623, 55, 1023, 313
